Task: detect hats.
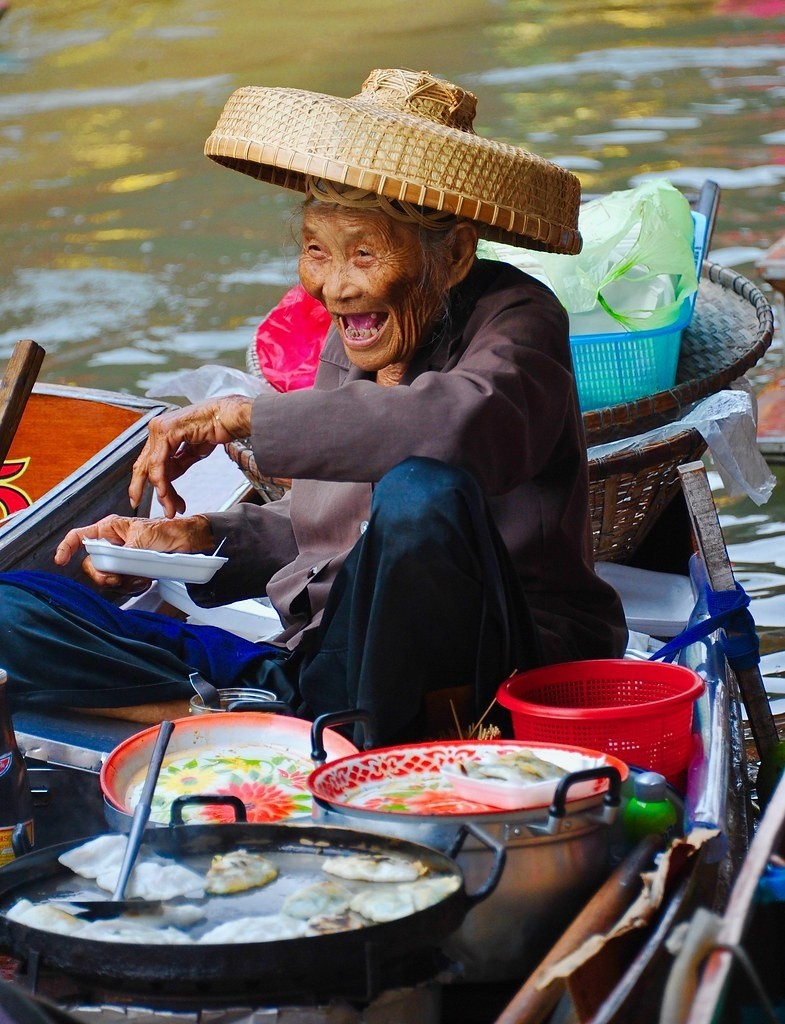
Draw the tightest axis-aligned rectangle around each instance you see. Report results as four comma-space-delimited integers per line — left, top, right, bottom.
202, 68, 584, 256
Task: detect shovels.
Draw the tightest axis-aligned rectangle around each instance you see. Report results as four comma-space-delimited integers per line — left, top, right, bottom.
47, 716, 176, 907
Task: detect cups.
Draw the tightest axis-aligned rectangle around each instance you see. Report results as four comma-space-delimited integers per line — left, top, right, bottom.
189, 688, 278, 715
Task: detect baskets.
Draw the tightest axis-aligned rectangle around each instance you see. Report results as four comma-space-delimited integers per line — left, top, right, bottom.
586, 378, 757, 564
565, 210, 707, 412
497, 659, 705, 775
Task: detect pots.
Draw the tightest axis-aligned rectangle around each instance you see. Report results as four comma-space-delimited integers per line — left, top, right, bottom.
0, 795, 507, 1006
312, 712, 622, 984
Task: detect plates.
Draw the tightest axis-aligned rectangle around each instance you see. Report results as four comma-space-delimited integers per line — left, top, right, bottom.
592, 562, 697, 638
81, 539, 229, 585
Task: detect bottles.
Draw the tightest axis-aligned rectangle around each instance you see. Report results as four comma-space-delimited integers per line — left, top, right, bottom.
623, 771, 677, 849
0, 669, 33, 870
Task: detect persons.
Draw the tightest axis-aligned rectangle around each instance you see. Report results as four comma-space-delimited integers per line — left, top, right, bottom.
1, 66, 629, 750
